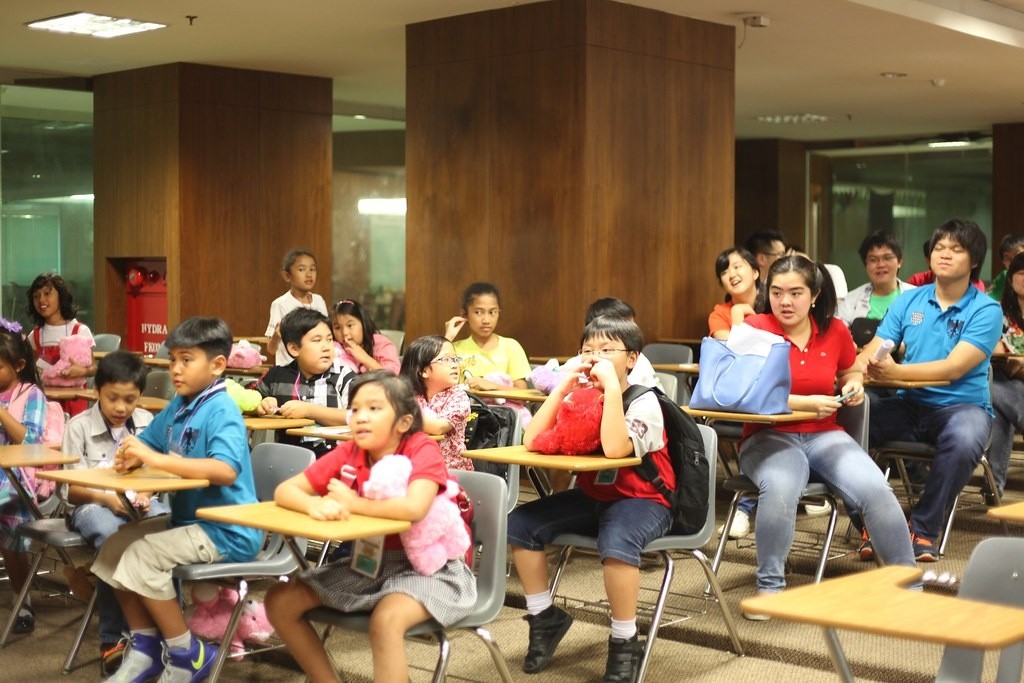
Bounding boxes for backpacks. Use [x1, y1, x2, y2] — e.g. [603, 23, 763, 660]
[9, 381, 65, 501]
[622, 385, 708, 535]
[227, 339, 268, 368]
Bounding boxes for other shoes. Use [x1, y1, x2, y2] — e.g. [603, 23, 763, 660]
[718, 511, 751, 538]
[12, 600, 35, 635]
[601, 629, 644, 683]
[98, 629, 129, 674]
[523, 606, 573, 673]
[806, 499, 835, 516]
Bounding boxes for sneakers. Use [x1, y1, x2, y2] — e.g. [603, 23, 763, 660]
[858, 527, 874, 560]
[102, 632, 164, 683]
[157, 636, 218, 683]
[908, 522, 940, 562]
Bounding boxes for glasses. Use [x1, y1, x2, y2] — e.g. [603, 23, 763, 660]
[577, 347, 632, 358]
[425, 355, 464, 366]
[762, 251, 784, 257]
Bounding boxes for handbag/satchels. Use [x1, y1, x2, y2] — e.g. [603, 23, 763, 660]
[464, 388, 515, 482]
[689, 337, 792, 416]
[849, 317, 885, 347]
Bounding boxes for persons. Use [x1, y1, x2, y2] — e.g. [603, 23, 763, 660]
[708, 218, 1024, 621]
[1, 250, 676, 683]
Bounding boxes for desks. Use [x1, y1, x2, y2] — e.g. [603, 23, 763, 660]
[243, 414, 315, 430]
[657, 337, 702, 345]
[93, 351, 143, 359]
[1, 443, 82, 468]
[43, 388, 91, 398]
[528, 355, 572, 363]
[142, 358, 170, 367]
[650, 362, 700, 373]
[475, 389, 547, 402]
[456, 444, 643, 472]
[233, 335, 269, 345]
[76, 387, 169, 410]
[739, 562, 1024, 650]
[286, 423, 445, 442]
[195, 500, 413, 544]
[34, 461, 209, 492]
[227, 365, 271, 374]
[681, 405, 818, 421]
[865, 379, 951, 388]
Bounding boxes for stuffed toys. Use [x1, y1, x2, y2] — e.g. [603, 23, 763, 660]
[226, 340, 268, 369]
[225, 379, 262, 416]
[525, 354, 586, 399]
[475, 374, 533, 431]
[361, 454, 471, 576]
[532, 376, 606, 456]
[181, 580, 275, 663]
[41, 335, 94, 388]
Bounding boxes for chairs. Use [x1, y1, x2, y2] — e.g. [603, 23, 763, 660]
[1, 331, 1023, 683]
